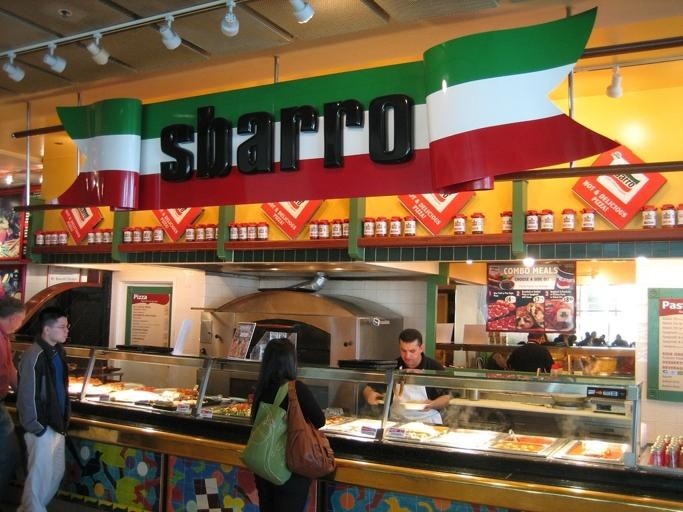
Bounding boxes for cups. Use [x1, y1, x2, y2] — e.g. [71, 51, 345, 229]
[554, 265, 574, 291]
[499, 280, 514, 290]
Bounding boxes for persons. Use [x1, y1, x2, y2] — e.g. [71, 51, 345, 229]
[361, 326, 454, 424]
[250, 337, 328, 512]
[0, 296, 28, 504]
[0, 217, 13, 243]
[15, 305, 72, 512]
[506, 331, 554, 373]
[486, 341, 509, 368]
[516, 327, 630, 348]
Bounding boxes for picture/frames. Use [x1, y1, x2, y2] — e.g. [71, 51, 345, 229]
[645, 286, 682, 404]
[124, 284, 172, 349]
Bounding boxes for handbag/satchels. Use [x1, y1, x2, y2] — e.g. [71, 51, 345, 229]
[239, 401, 292, 486]
[286, 399, 337, 479]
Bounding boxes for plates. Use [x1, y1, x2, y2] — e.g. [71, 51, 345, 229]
[488, 276, 514, 284]
[399, 402, 430, 410]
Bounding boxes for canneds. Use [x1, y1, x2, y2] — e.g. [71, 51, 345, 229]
[34, 230, 68, 247]
[123, 226, 164, 243]
[452, 214, 468, 235]
[363, 216, 416, 237]
[525, 208, 595, 232]
[640, 204, 683, 229]
[185, 223, 219, 242]
[87, 229, 113, 245]
[471, 213, 484, 234]
[500, 211, 512, 233]
[229, 222, 269, 241]
[309, 218, 350, 239]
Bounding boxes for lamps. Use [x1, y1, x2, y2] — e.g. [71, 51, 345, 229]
[605, 65, 623, 98]
[0, 0, 314, 83]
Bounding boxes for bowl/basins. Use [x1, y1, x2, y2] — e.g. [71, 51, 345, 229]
[551, 391, 587, 407]
